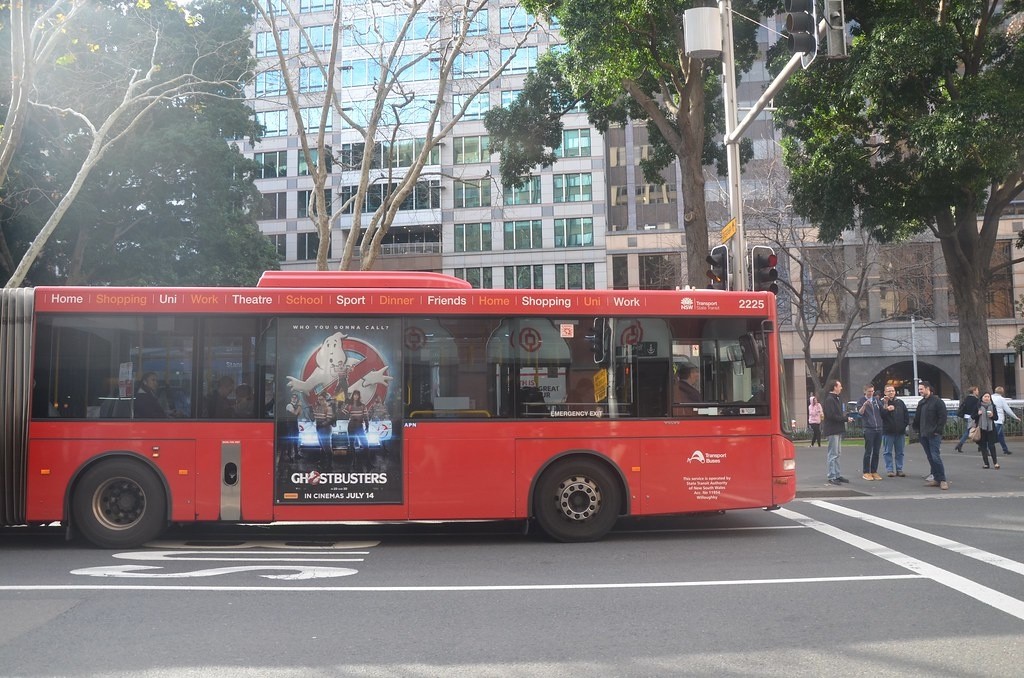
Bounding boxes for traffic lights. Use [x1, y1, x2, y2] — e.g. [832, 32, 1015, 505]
[706, 252, 728, 290]
[757, 251, 779, 296]
[782, 0, 815, 55]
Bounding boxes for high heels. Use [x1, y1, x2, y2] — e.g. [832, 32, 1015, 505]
[977, 446, 981, 452]
[955, 442, 963, 452]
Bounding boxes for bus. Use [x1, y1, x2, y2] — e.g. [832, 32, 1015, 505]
[1, 269, 799, 550]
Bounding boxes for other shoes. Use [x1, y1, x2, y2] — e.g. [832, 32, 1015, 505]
[830, 479, 841, 486]
[939, 480, 948, 490]
[896, 470, 906, 477]
[819, 412, 825, 422]
[808, 444, 814, 448]
[994, 463, 1000, 468]
[928, 480, 939, 486]
[862, 473, 874, 481]
[871, 472, 882, 480]
[1003, 450, 1013, 456]
[887, 471, 895, 476]
[836, 476, 849, 483]
[983, 464, 991, 469]
[922, 474, 934, 482]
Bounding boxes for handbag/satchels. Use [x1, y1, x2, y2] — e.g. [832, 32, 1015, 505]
[957, 400, 967, 418]
[968, 425, 981, 443]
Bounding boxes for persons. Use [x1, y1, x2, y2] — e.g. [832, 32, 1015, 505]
[313, 395, 334, 473]
[955, 386, 980, 453]
[857, 384, 889, 481]
[206, 376, 254, 418]
[678, 363, 703, 403]
[135, 372, 164, 419]
[971, 392, 1001, 469]
[809, 397, 824, 448]
[366, 395, 391, 454]
[911, 381, 949, 490]
[336, 390, 375, 469]
[990, 387, 1021, 454]
[821, 380, 854, 485]
[285, 394, 302, 462]
[880, 384, 909, 478]
